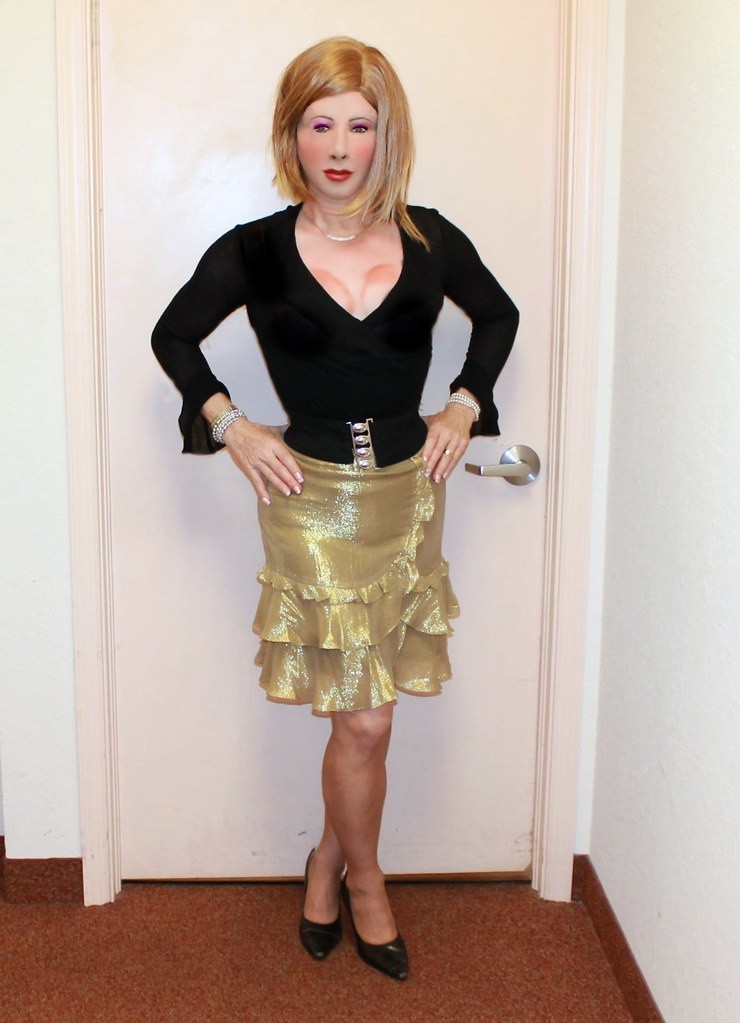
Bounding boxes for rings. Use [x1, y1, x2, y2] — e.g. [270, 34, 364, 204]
[443, 449, 454, 457]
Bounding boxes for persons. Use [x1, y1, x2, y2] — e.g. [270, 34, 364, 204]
[152, 38, 519, 982]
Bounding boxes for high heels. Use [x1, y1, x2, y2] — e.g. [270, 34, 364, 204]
[342, 870, 409, 982]
[299, 848, 343, 961]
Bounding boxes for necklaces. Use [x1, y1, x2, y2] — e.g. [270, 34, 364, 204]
[300, 208, 377, 241]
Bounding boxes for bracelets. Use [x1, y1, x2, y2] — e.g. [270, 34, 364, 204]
[210, 404, 246, 444]
[445, 392, 480, 421]
